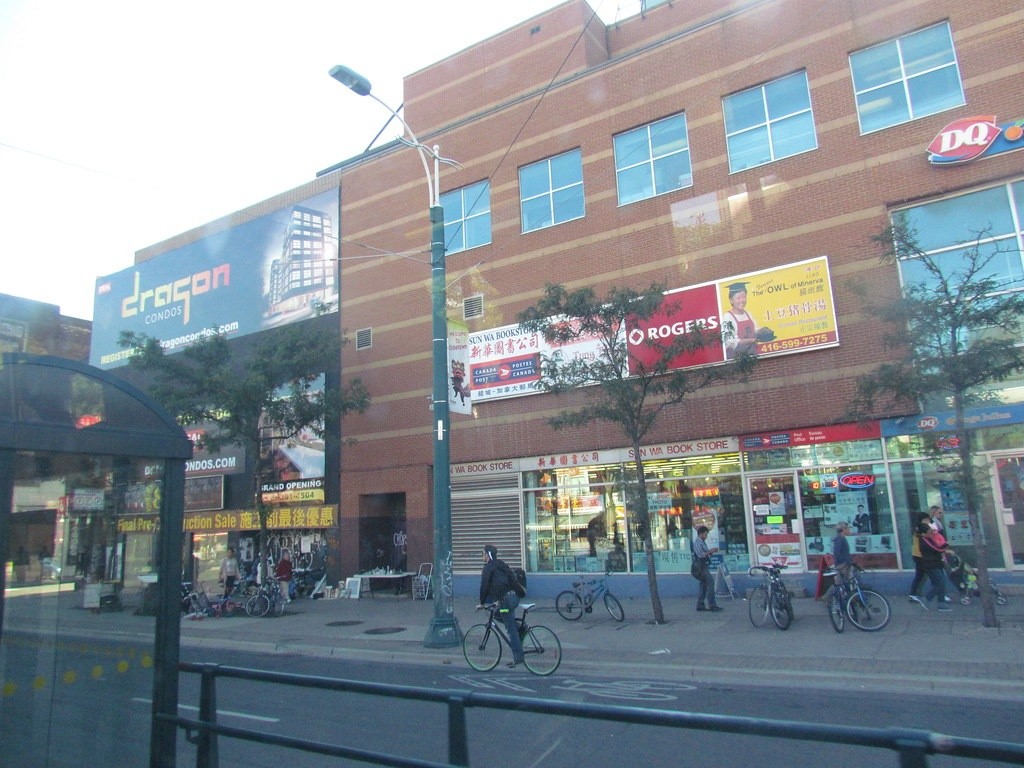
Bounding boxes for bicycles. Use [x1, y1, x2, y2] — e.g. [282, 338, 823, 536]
[820, 561, 891, 633]
[181, 581, 246, 620]
[244, 574, 289, 618]
[555, 568, 625, 623]
[463, 604, 563, 677]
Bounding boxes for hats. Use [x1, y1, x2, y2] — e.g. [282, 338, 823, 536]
[725, 280, 749, 297]
[928, 522, 937, 530]
[483, 544, 497, 561]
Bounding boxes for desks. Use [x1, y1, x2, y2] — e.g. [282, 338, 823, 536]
[353, 568, 416, 600]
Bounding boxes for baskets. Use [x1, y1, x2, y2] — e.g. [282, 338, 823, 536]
[856, 572, 877, 591]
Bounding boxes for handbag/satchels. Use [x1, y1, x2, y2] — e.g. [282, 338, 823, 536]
[512, 568, 526, 598]
[691, 560, 707, 580]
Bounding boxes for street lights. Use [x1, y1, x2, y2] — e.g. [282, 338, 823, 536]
[327, 64, 465, 649]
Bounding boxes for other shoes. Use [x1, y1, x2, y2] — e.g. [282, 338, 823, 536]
[938, 602, 953, 612]
[944, 594, 951, 602]
[506, 659, 524, 667]
[696, 607, 710, 612]
[909, 594, 922, 603]
[711, 606, 724, 611]
[519, 626, 526, 640]
[920, 597, 931, 611]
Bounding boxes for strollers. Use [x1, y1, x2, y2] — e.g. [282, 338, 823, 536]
[942, 550, 1008, 606]
[748, 565, 795, 632]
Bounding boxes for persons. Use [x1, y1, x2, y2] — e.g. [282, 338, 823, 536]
[276, 552, 293, 604]
[853, 505, 870, 534]
[12, 548, 52, 583]
[722, 282, 760, 359]
[218, 548, 241, 602]
[252, 553, 261, 589]
[819, 522, 852, 607]
[694, 527, 721, 611]
[477, 545, 529, 667]
[910, 507, 953, 612]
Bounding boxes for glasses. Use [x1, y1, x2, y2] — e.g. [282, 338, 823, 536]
[938, 511, 942, 515]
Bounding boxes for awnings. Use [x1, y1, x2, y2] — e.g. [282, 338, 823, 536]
[526, 513, 597, 530]
